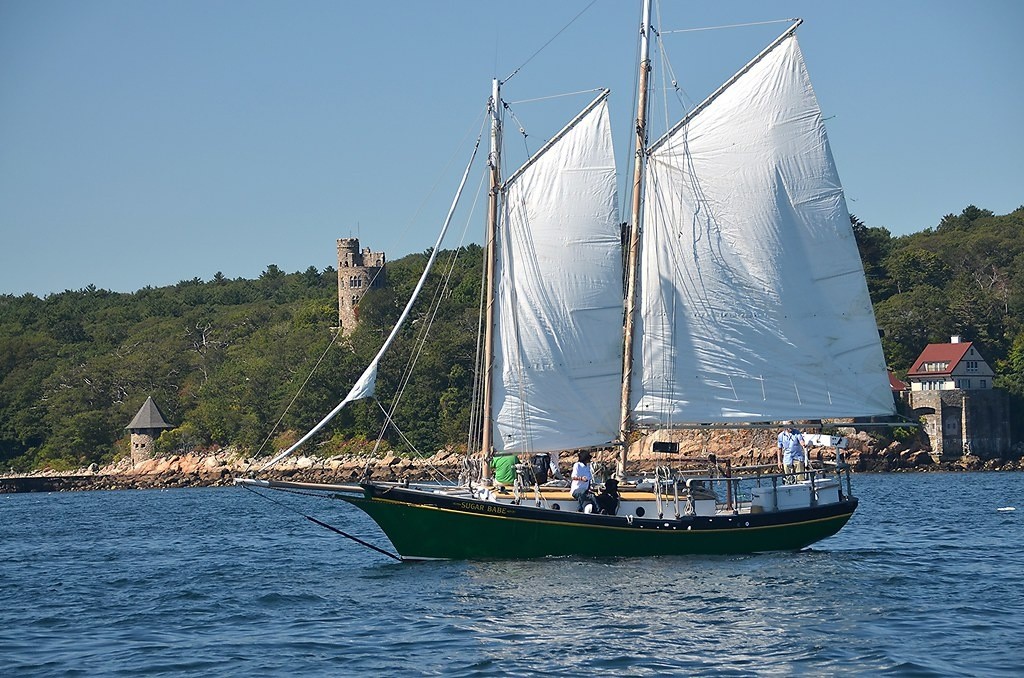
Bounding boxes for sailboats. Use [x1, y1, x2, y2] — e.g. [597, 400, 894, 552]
[228, 0, 918, 565]
[432, 472, 717, 513]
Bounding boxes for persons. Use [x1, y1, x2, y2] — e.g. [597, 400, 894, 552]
[777, 420, 808, 485]
[548, 452, 563, 481]
[490, 453, 521, 487]
[571, 450, 606, 515]
